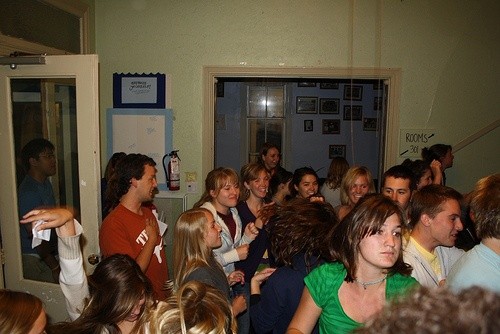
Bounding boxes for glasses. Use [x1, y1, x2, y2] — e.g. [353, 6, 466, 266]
[40, 154, 56, 160]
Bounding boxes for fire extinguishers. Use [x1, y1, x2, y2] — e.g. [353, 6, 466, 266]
[161, 148, 182, 192]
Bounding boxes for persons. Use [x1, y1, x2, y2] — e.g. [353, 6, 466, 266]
[286, 192, 420, 334]
[251, 202, 339, 334]
[195, 167, 258, 277]
[380, 165, 414, 209]
[270, 171, 292, 201]
[98, 153, 168, 301]
[293, 166, 324, 201]
[173, 208, 246, 334]
[412, 159, 433, 189]
[0, 289, 47, 334]
[446, 174, 500, 299]
[256, 145, 287, 179]
[454, 191, 482, 251]
[18, 139, 63, 282]
[137, 279, 237, 334]
[47, 254, 152, 334]
[421, 144, 454, 187]
[337, 166, 375, 216]
[404, 184, 469, 296]
[321, 156, 351, 206]
[235, 163, 275, 246]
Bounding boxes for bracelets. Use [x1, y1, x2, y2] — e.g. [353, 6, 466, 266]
[52, 264, 60, 272]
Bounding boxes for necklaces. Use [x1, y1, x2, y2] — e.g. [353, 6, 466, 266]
[353, 273, 388, 288]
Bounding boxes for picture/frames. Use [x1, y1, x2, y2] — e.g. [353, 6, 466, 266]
[294, 82, 381, 159]
[106, 109, 173, 191]
